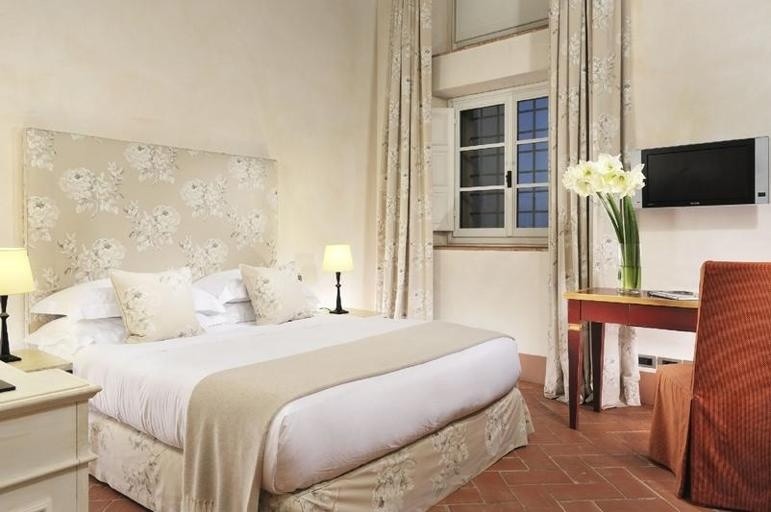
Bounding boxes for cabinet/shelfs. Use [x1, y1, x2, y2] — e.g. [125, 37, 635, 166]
[0, 360, 103, 512]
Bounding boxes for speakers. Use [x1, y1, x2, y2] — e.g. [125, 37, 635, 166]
[755, 135, 770, 205]
[630, 149, 642, 209]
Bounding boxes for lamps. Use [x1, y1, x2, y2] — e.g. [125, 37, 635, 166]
[0, 247, 37, 362]
[323, 243, 351, 314]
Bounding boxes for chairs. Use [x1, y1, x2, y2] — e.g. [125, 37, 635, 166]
[645, 261, 771, 512]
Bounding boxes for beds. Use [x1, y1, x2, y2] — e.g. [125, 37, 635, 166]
[20, 126, 534, 512]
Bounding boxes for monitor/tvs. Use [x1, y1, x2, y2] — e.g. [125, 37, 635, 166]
[641, 137, 756, 209]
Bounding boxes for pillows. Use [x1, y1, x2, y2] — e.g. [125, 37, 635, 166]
[24, 314, 223, 347]
[107, 269, 203, 342]
[238, 262, 321, 327]
[192, 268, 251, 303]
[224, 299, 257, 323]
[29, 274, 224, 322]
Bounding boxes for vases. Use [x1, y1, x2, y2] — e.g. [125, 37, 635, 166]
[616, 241, 642, 298]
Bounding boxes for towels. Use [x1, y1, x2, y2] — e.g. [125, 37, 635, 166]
[181, 319, 513, 512]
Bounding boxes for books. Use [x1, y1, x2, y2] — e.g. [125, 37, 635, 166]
[647, 289, 699, 301]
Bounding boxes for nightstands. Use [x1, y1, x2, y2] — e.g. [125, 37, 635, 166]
[10, 347, 73, 374]
[337, 308, 387, 316]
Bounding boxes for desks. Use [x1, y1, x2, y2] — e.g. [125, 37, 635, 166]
[562, 288, 699, 429]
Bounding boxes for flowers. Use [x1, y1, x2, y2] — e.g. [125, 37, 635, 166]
[561, 152, 647, 290]
